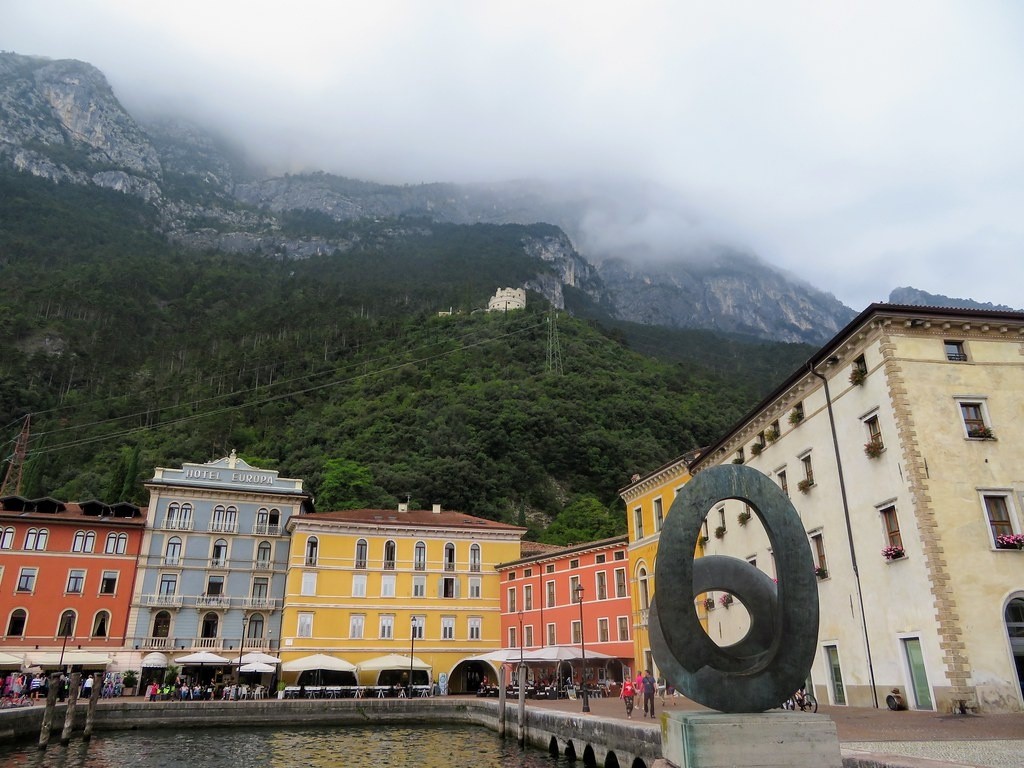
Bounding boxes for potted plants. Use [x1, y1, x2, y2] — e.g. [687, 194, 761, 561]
[863, 442, 883, 459]
[787, 409, 804, 427]
[698, 536, 709, 548]
[750, 442, 765, 455]
[715, 527, 726, 538]
[277, 679, 288, 699]
[764, 428, 780, 443]
[798, 479, 813, 495]
[121, 670, 139, 695]
[849, 368, 866, 387]
[737, 512, 750, 527]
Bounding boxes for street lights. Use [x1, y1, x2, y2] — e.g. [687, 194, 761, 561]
[408, 615, 418, 699]
[516, 608, 524, 664]
[574, 584, 591, 712]
[234, 615, 249, 701]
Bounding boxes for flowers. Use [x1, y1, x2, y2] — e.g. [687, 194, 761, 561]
[881, 545, 905, 560]
[703, 598, 713, 611]
[815, 565, 827, 578]
[772, 578, 778, 583]
[971, 425, 992, 437]
[997, 534, 1024, 550]
[717, 594, 732, 609]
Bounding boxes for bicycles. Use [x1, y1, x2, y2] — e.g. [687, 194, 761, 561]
[0, 682, 34, 710]
[781, 689, 818, 713]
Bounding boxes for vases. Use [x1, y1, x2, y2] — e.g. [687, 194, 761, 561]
[999, 543, 1017, 549]
[728, 600, 733, 603]
[968, 431, 991, 438]
[710, 604, 714, 608]
[821, 571, 828, 579]
[891, 552, 904, 559]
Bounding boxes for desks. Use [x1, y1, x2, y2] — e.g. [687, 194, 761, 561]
[477, 684, 602, 700]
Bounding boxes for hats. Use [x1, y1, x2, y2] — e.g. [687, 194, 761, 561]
[88, 675, 93, 678]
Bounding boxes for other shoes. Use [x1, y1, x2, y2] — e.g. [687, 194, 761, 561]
[634, 705, 639, 710]
[36, 699, 40, 701]
[651, 715, 655, 718]
[644, 712, 647, 717]
[628, 716, 631, 719]
[642, 708, 644, 710]
[662, 700, 665, 706]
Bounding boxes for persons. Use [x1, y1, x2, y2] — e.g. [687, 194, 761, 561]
[0, 672, 263, 701]
[477, 669, 679, 718]
[795, 690, 807, 711]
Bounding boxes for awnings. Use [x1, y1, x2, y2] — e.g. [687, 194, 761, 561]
[0, 653, 25, 665]
[24, 652, 112, 668]
[141, 652, 168, 668]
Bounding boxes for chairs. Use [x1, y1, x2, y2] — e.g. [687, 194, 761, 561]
[240, 684, 270, 700]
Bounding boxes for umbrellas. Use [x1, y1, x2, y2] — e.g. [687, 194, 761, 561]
[237, 661, 276, 673]
[173, 651, 231, 666]
[506, 645, 617, 697]
[230, 651, 282, 665]
[468, 650, 531, 664]
[356, 654, 432, 671]
[281, 653, 358, 686]
